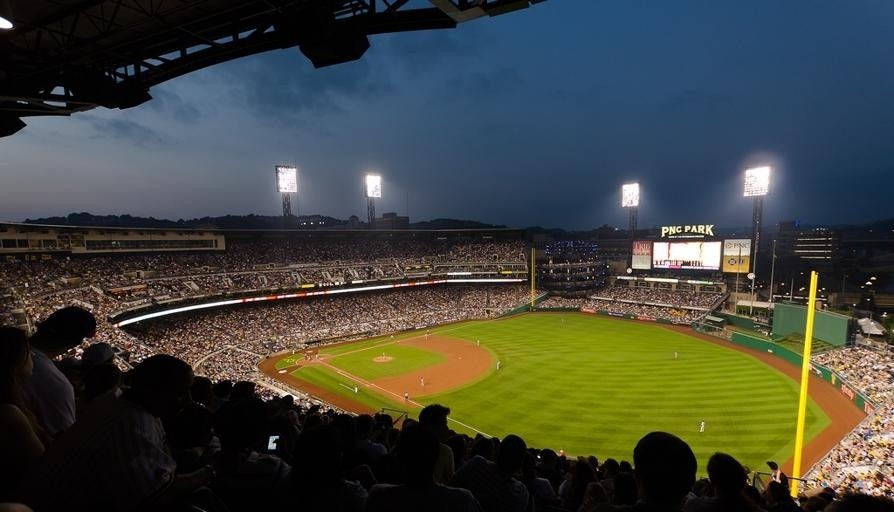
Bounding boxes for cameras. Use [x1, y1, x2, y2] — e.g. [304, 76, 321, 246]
[267, 435, 280, 451]
